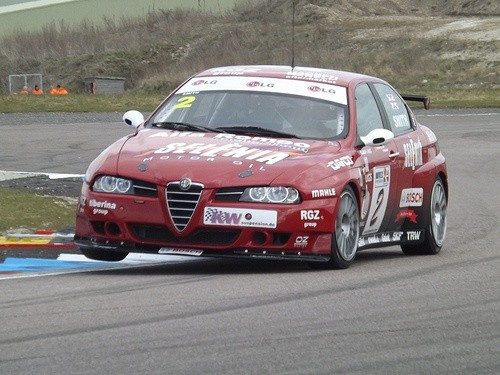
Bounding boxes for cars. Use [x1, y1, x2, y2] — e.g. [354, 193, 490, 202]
[74, 64, 449, 270]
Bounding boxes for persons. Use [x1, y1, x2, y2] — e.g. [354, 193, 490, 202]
[298, 105, 332, 137]
